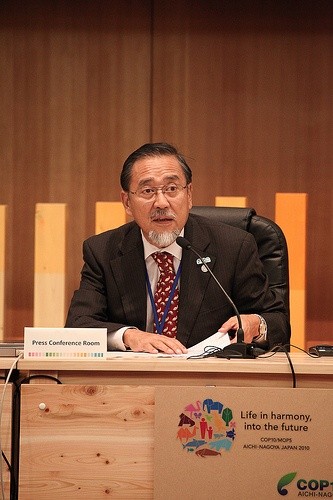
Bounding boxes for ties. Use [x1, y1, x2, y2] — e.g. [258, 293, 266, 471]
[152, 250, 181, 339]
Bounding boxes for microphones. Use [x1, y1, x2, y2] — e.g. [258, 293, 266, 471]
[176, 236, 257, 359]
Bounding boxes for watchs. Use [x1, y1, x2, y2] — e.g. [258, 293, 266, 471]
[251, 314, 267, 342]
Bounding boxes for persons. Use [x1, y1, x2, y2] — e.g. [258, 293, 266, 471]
[64, 143, 292, 354]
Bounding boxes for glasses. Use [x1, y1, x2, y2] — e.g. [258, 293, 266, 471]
[126, 183, 188, 197]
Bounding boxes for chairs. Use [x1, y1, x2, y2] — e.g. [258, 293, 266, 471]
[190, 206, 291, 353]
